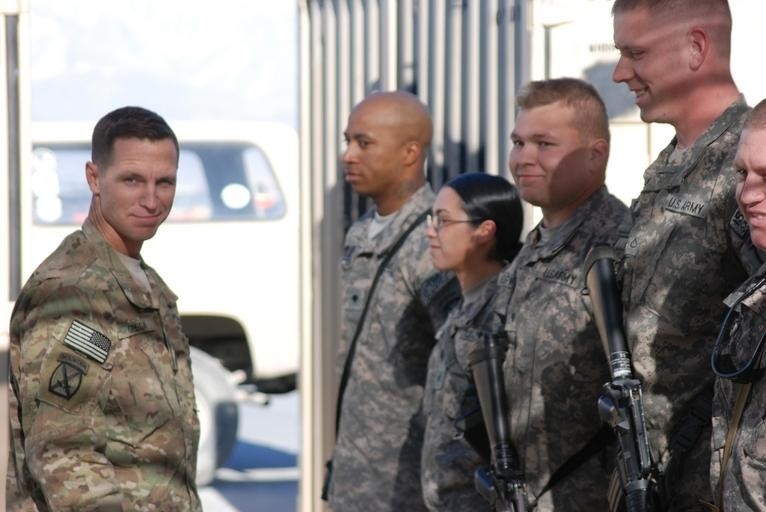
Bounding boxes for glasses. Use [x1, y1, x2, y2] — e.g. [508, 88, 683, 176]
[426, 214, 472, 230]
[711, 278, 766, 384]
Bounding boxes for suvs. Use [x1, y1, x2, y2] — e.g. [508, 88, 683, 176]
[19, 114, 299, 488]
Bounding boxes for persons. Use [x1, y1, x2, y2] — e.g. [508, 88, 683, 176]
[608, 0, 766, 512]
[327, 90, 462, 512]
[490, 79, 628, 512]
[420, 174, 523, 512]
[710, 99, 766, 512]
[5, 107, 203, 512]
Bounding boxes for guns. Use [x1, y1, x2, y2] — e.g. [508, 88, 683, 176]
[467, 333, 530, 511]
[581, 243, 658, 511]
